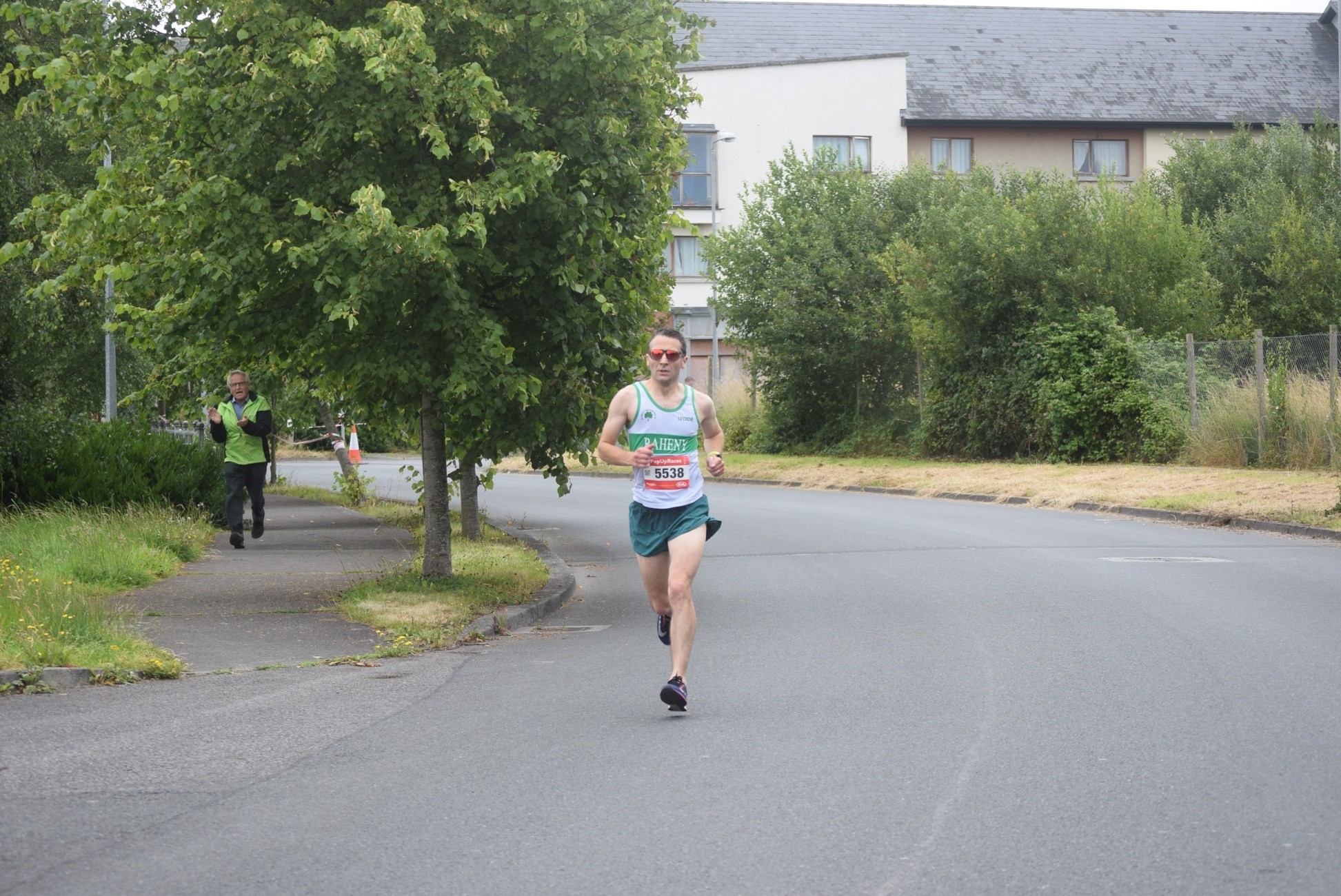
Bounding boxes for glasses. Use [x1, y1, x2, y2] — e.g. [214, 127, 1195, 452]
[648, 349, 682, 361]
[228, 382, 246, 387]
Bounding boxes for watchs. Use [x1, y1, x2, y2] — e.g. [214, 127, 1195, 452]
[708, 451, 722, 459]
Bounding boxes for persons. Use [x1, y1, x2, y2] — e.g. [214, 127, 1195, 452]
[209, 370, 272, 549]
[598, 330, 726, 711]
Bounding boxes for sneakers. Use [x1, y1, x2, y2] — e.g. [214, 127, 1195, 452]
[251, 518, 264, 538]
[230, 531, 244, 548]
[660, 675, 688, 712]
[657, 615, 671, 645]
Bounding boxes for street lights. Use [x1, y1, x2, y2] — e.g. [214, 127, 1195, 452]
[708, 135, 738, 407]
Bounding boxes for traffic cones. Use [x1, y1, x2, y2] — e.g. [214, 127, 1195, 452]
[348, 425, 367, 468]
